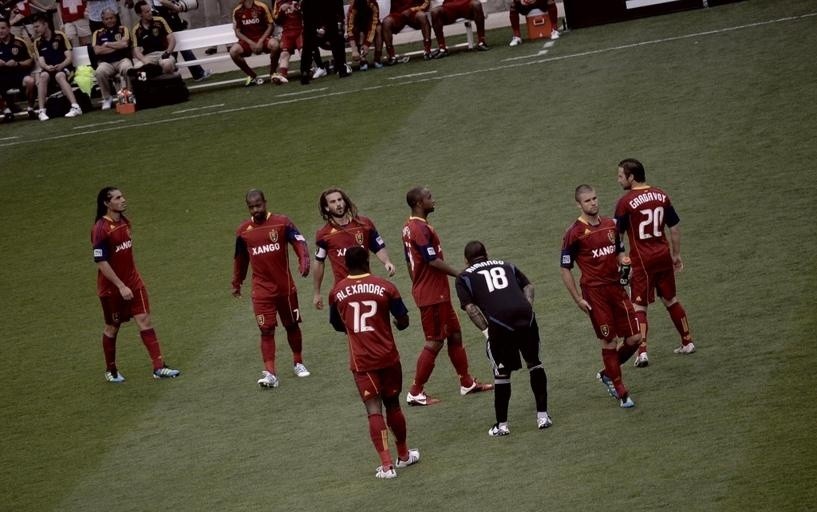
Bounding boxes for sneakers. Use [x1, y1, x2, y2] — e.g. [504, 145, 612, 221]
[673, 342, 696, 354]
[476, 41, 490, 51]
[633, 351, 649, 367]
[488, 421, 511, 437]
[386, 56, 397, 65]
[432, 48, 448, 59]
[395, 448, 420, 468]
[244, 71, 257, 87]
[617, 391, 635, 408]
[596, 367, 618, 401]
[152, 366, 181, 379]
[2, 113, 16, 123]
[270, 73, 288, 85]
[343, 63, 352, 74]
[460, 377, 494, 396]
[406, 391, 440, 406]
[193, 71, 211, 82]
[104, 370, 127, 383]
[423, 50, 433, 61]
[301, 70, 309, 85]
[65, 107, 83, 117]
[359, 64, 368, 71]
[509, 36, 522, 47]
[535, 415, 553, 429]
[102, 95, 113, 110]
[38, 111, 50, 121]
[257, 370, 279, 388]
[375, 465, 398, 479]
[374, 61, 383, 68]
[293, 362, 311, 378]
[312, 66, 327, 79]
[28, 111, 36, 120]
[550, 28, 559, 40]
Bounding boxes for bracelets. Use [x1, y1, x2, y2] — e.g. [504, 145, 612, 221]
[576, 296, 583, 304]
[481, 326, 491, 341]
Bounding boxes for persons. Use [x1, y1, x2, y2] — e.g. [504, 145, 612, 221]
[230, 1, 561, 88]
[311, 187, 395, 311]
[453, 240, 553, 438]
[400, 186, 494, 406]
[558, 183, 642, 407]
[229, 189, 311, 388]
[88, 186, 180, 384]
[0, 0, 233, 122]
[328, 246, 420, 480]
[608, 158, 696, 367]
